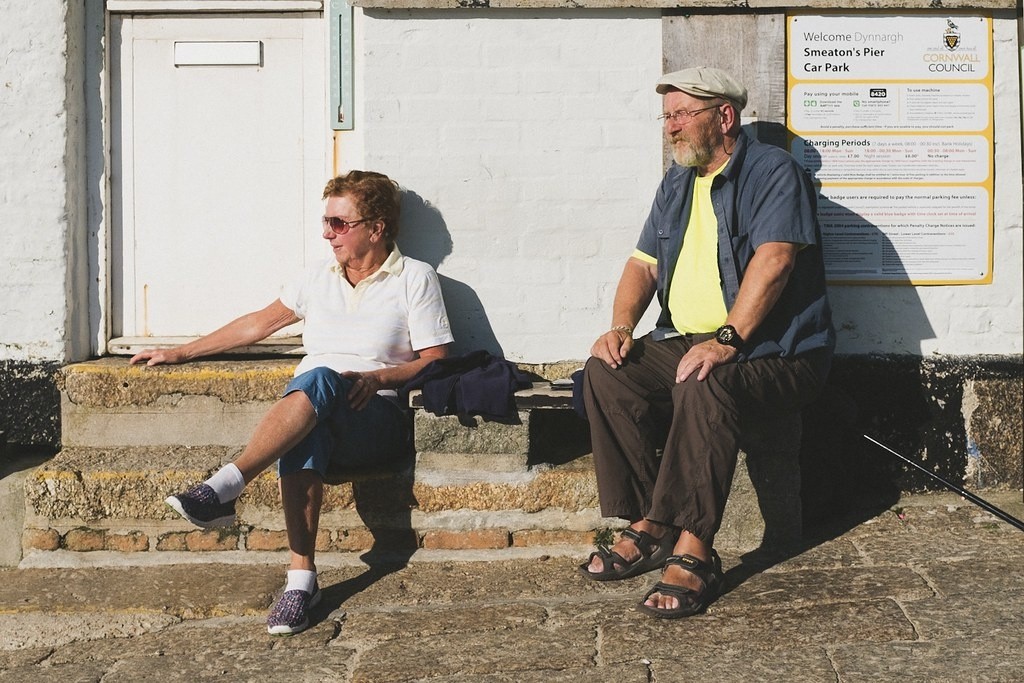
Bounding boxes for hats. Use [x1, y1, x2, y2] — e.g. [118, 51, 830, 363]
[655, 66, 748, 112]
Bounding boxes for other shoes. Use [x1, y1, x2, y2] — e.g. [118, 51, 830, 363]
[164, 481, 237, 528]
[267, 575, 321, 634]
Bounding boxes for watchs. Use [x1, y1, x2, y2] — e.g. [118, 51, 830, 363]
[715, 325, 745, 351]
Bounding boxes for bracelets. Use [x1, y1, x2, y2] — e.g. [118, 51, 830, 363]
[610, 326, 632, 336]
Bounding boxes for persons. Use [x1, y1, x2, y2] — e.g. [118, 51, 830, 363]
[578, 64, 838, 619]
[129, 168, 454, 635]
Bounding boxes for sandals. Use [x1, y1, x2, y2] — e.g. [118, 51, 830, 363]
[636, 546, 727, 620]
[581, 527, 678, 581]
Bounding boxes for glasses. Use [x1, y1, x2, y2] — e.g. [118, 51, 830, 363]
[321, 215, 379, 234]
[658, 104, 724, 126]
